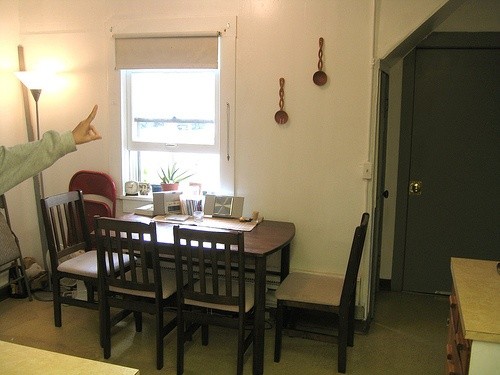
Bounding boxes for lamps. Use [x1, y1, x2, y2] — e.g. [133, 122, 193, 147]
[17, 71, 55, 300]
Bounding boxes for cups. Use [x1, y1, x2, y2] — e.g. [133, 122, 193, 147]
[194, 211, 204, 223]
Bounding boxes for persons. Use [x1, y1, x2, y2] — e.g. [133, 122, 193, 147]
[0, 104, 102, 197]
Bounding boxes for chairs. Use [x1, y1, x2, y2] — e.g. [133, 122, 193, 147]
[42, 171, 253, 375]
[0, 195, 33, 301]
[275, 212, 370, 372]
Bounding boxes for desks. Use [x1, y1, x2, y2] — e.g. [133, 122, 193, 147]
[90, 213, 296, 375]
[0, 340, 140, 375]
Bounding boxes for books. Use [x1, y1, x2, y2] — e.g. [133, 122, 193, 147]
[133, 204, 153, 216]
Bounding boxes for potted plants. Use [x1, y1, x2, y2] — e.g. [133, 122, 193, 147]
[158, 160, 194, 191]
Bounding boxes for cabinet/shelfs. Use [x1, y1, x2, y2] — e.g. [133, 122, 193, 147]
[445, 257, 500, 375]
